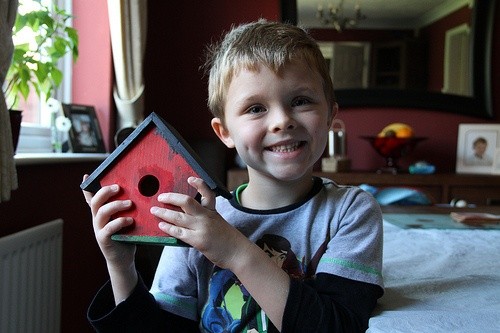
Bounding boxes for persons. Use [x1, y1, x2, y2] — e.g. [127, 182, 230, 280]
[465, 137, 493, 167]
[80, 20, 384, 333]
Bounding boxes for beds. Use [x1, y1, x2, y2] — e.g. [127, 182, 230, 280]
[228, 169, 500, 333]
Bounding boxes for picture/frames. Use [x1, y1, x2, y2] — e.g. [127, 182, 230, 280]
[60, 103, 107, 153]
[456, 123, 500, 174]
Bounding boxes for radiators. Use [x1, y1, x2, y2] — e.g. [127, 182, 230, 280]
[0, 218, 63, 333]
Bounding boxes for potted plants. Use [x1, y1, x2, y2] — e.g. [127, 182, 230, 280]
[4, 0, 80, 154]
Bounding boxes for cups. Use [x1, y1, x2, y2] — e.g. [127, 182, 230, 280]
[329, 128, 348, 158]
